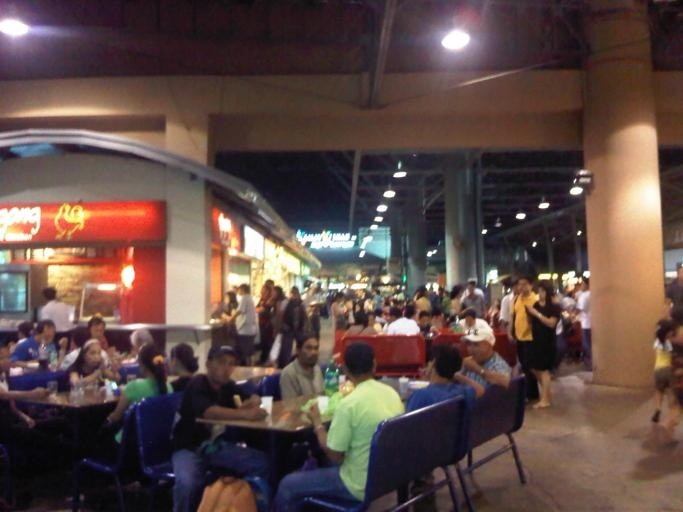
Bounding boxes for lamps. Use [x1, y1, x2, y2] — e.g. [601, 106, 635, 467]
[357, 161, 593, 258]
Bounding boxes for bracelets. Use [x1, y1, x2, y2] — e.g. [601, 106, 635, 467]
[310, 424, 326, 435]
[479, 367, 486, 377]
[537, 313, 542, 319]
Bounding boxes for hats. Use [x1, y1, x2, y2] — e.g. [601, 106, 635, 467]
[460, 328, 496, 346]
[208, 346, 238, 361]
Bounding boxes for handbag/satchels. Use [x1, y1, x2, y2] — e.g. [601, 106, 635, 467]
[562, 318, 576, 336]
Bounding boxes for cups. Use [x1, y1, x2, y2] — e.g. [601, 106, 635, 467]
[71, 380, 87, 400]
[399, 378, 408, 398]
[46, 378, 56, 396]
[260, 394, 272, 416]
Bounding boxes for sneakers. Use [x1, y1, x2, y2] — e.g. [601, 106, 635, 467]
[533, 399, 551, 408]
[652, 410, 660, 422]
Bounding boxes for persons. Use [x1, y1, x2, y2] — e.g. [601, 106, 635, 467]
[211, 274, 591, 411]
[407, 345, 469, 413]
[648, 318, 677, 425]
[452, 321, 518, 396]
[2, 285, 199, 512]
[167, 343, 272, 512]
[665, 261, 683, 331]
[273, 340, 406, 512]
[279, 332, 327, 402]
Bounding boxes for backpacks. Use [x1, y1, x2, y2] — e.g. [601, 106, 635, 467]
[197, 477, 257, 511]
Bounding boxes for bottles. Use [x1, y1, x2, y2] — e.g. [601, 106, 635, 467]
[453, 315, 462, 333]
[323, 360, 339, 394]
[37, 339, 50, 368]
